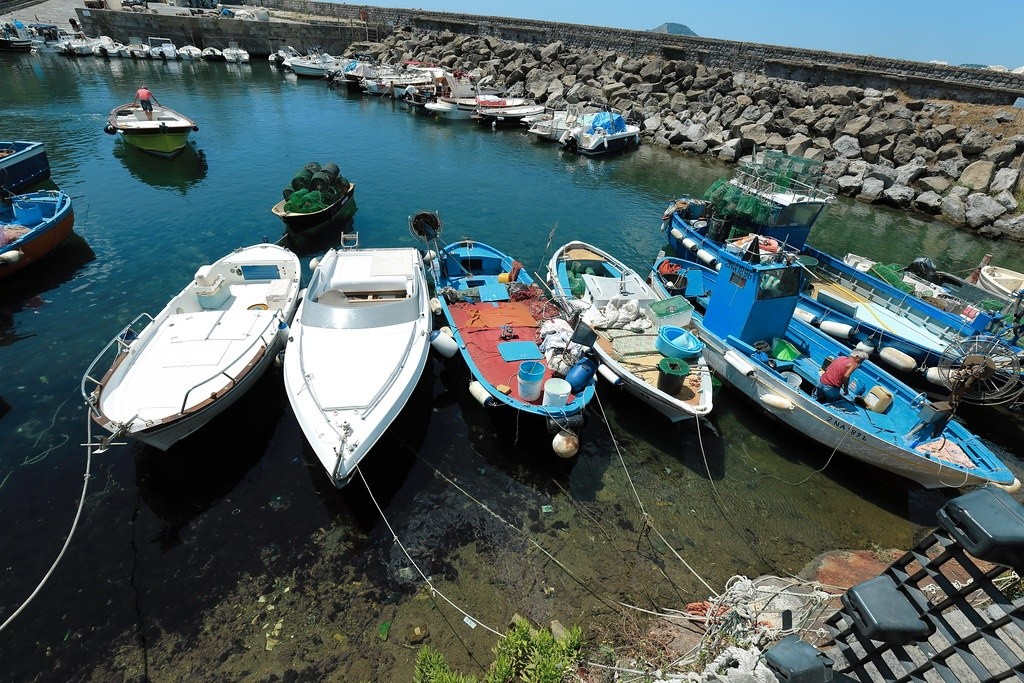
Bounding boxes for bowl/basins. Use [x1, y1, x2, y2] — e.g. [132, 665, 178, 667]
[656, 325, 705, 360]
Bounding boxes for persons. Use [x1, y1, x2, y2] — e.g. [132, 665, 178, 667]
[134, 85, 162, 121]
[812, 349, 870, 403]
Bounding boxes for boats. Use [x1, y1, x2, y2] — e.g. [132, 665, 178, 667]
[268, 46, 642, 155]
[201, 47, 223, 61]
[660, 193, 1024, 408]
[0, 139, 51, 193]
[908, 257, 1024, 321]
[546, 241, 713, 423]
[271, 180, 356, 228]
[179, 44, 202, 60]
[149, 37, 181, 60]
[964, 254, 1024, 305]
[646, 249, 1021, 494]
[118, 35, 151, 58]
[428, 236, 596, 426]
[0, 19, 125, 57]
[843, 251, 1023, 349]
[0, 189, 75, 275]
[276, 232, 433, 488]
[79, 233, 301, 452]
[107, 102, 200, 151]
[221, 40, 250, 62]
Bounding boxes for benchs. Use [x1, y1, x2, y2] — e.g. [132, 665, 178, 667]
[330, 275, 408, 303]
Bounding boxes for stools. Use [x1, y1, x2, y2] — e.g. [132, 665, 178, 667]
[266, 278, 292, 310]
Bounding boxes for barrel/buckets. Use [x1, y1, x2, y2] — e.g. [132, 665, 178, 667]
[661, 273, 688, 297]
[671, 332, 694, 349]
[864, 385, 893, 412]
[848, 376, 866, 394]
[781, 371, 802, 390]
[656, 356, 691, 396]
[678, 199, 705, 219]
[517, 357, 597, 407]
[920, 294, 969, 317]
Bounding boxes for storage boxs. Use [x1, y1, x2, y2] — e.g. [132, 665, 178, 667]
[646, 295, 694, 332]
[194, 278, 231, 309]
[195, 265, 218, 286]
